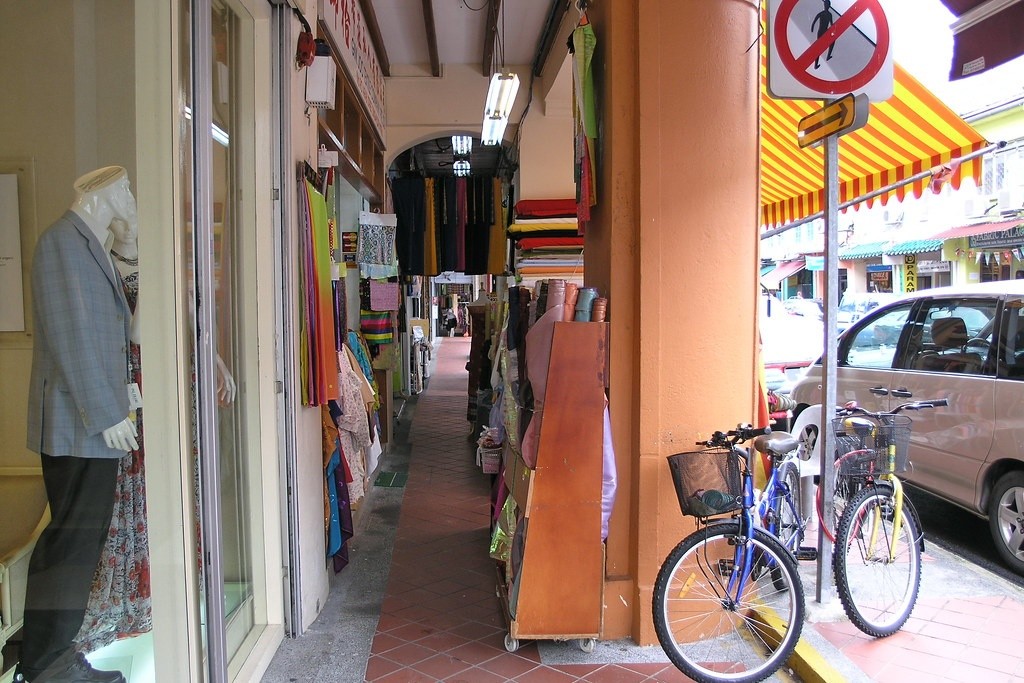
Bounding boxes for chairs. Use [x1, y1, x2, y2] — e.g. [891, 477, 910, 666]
[915, 316, 982, 374]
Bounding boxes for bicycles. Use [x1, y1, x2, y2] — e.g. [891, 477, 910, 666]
[831, 397, 949, 638]
[651, 425, 820, 683]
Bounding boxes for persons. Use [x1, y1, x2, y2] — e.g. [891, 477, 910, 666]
[13, 165, 236, 683]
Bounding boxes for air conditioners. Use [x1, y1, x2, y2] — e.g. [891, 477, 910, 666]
[998, 187, 1024, 215]
[882, 206, 903, 225]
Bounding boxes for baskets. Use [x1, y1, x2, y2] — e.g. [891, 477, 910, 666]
[480, 445, 502, 474]
[831, 413, 913, 475]
[666, 449, 744, 517]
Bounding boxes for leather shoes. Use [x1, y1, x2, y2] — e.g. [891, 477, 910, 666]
[12, 643, 126, 683]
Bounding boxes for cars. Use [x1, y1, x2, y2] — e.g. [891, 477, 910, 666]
[760, 291, 996, 423]
[788, 278, 1024, 579]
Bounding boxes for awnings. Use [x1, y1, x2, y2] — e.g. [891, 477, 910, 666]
[760, 260, 806, 290]
[759, 0, 1024, 231]
[839, 238, 944, 260]
[925, 219, 1023, 241]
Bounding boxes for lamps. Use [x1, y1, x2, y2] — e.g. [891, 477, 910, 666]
[479, 0, 520, 147]
[451, 135, 472, 155]
[452, 157, 472, 178]
